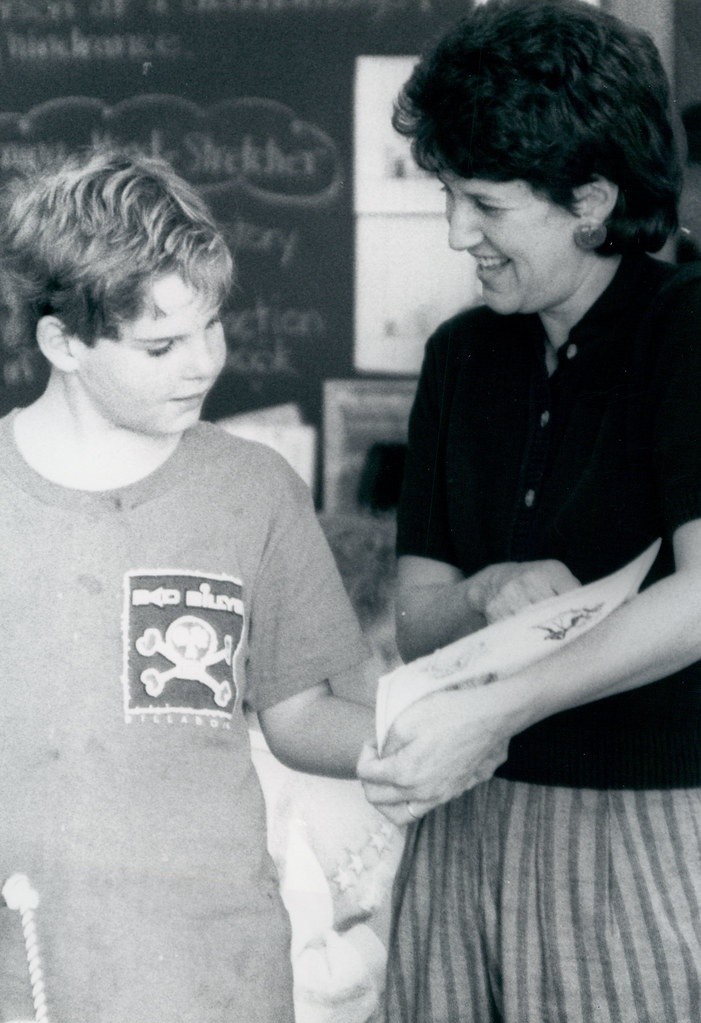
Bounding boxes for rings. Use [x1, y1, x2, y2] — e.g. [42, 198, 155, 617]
[407, 802, 423, 820]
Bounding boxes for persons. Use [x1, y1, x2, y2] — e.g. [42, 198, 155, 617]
[0, 154, 507, 1023]
[353, 0, 700, 1022]
